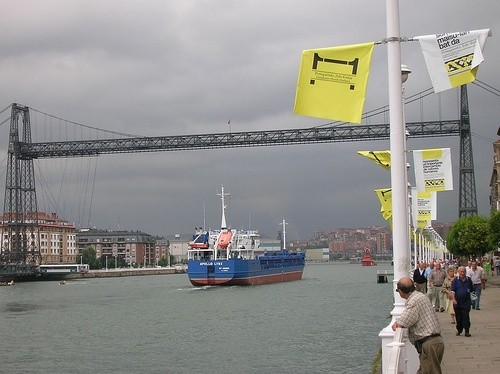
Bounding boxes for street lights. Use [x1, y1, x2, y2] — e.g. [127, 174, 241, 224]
[378, 2, 454, 373]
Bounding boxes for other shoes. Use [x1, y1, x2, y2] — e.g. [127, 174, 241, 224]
[455, 331, 460, 335]
[465, 333, 471, 336]
[435, 307, 439, 311]
[451, 320, 455, 324]
[440, 307, 444, 312]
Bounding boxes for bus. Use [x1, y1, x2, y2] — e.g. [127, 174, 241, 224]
[39, 264, 90, 273]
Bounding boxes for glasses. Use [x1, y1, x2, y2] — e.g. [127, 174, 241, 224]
[396, 289, 398, 291]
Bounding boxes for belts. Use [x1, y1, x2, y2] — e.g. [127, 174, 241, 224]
[433, 284, 441, 286]
[473, 283, 481, 285]
[414, 333, 440, 344]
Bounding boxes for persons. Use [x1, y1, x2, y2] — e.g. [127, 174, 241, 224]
[468, 261, 482, 310]
[429, 262, 446, 312]
[451, 267, 473, 337]
[392, 277, 444, 374]
[441, 267, 454, 324]
[424, 262, 435, 308]
[413, 263, 426, 294]
[409, 257, 500, 290]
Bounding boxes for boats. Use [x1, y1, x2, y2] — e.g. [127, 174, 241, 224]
[361, 247, 379, 266]
[186, 184, 306, 287]
